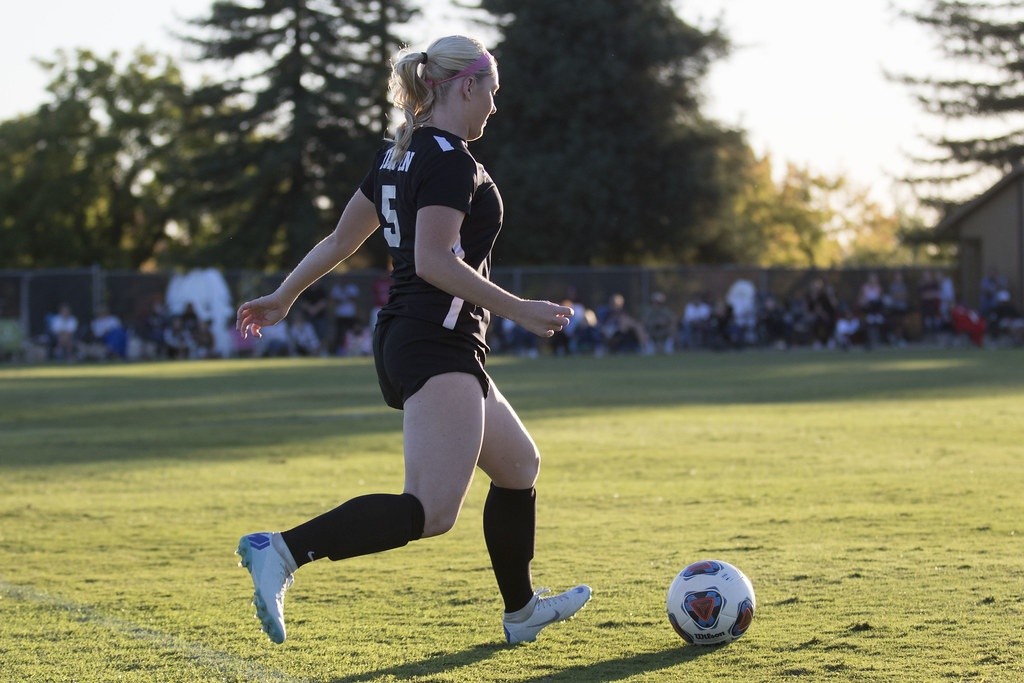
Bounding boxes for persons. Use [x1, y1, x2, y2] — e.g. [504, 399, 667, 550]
[236, 35, 591, 645]
[47, 271, 1024, 368]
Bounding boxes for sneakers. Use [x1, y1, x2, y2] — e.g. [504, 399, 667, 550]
[502, 585, 592, 645]
[234, 531, 296, 645]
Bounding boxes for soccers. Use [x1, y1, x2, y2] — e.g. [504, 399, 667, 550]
[668, 561, 757, 648]
